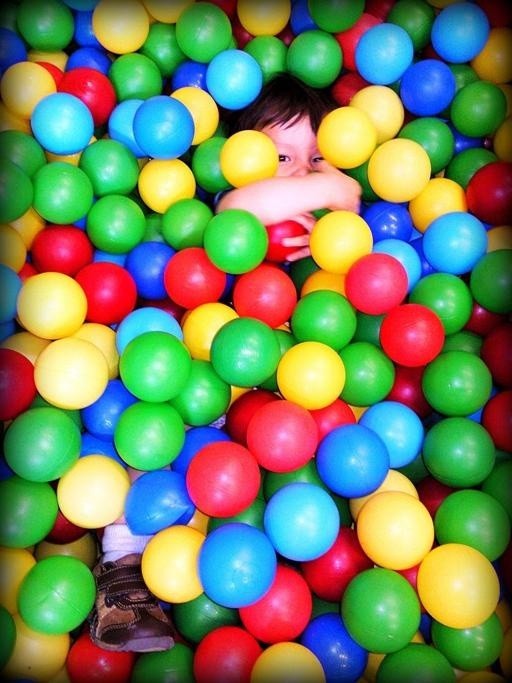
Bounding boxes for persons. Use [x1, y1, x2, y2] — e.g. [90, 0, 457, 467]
[88, 80, 368, 653]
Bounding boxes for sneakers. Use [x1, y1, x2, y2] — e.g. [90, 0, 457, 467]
[89, 555, 175, 653]
